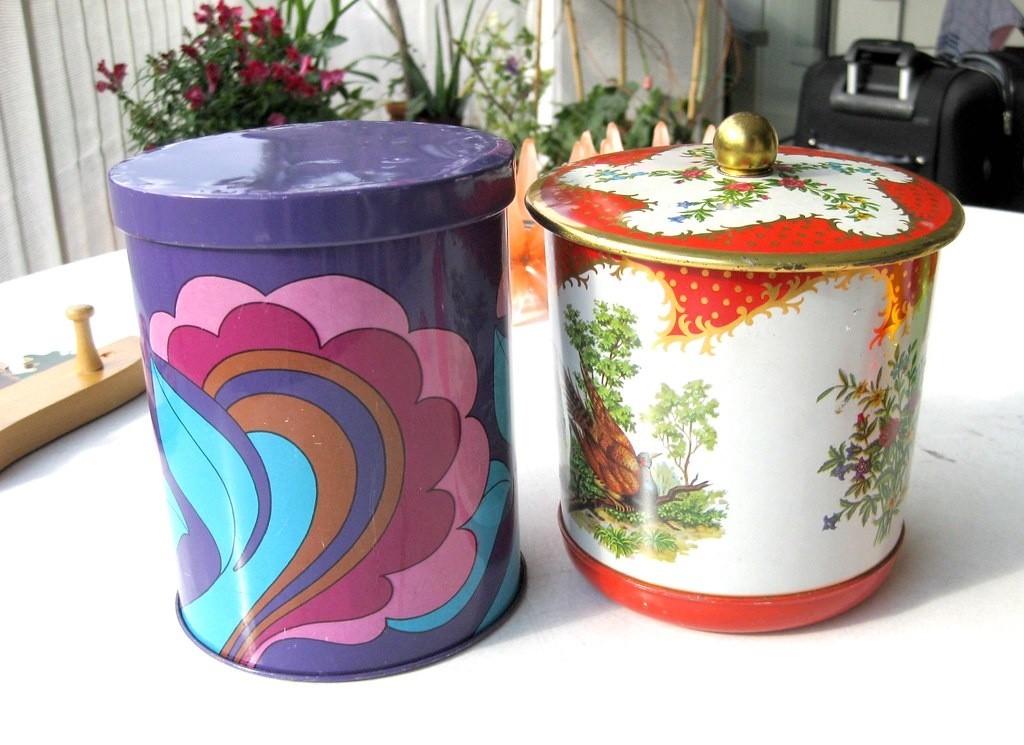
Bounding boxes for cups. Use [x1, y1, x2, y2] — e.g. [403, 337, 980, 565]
[106, 118, 530, 684]
[524, 111, 966, 636]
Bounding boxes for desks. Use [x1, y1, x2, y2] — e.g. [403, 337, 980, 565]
[0, 204, 1024, 745]
[723, 30, 769, 120]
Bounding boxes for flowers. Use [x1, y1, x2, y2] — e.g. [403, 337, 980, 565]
[96, 0, 717, 311]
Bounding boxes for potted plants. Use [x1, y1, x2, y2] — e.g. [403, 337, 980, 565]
[383, 97, 464, 127]
